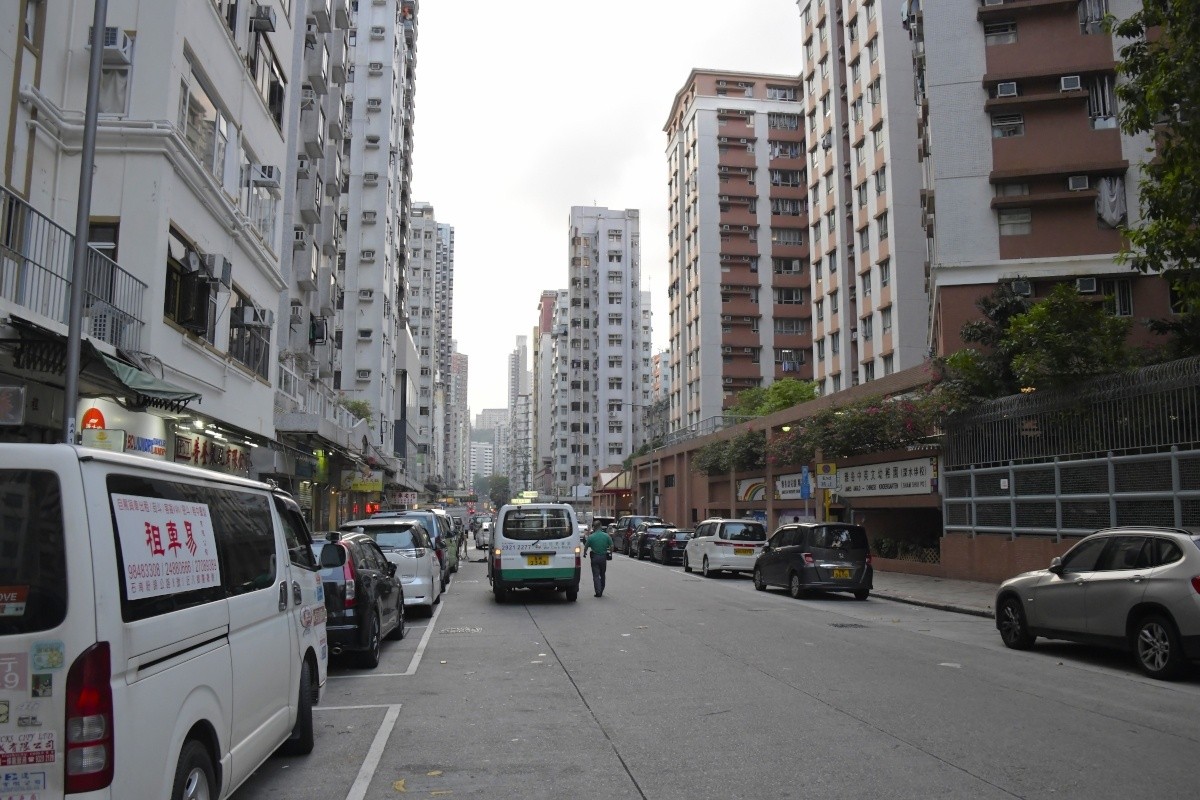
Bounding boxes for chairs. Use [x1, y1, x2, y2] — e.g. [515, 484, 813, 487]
[1125, 548, 1148, 568]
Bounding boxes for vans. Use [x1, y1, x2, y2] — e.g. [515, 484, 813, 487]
[609, 515, 663, 556]
[681, 516, 768, 577]
[486, 502, 582, 604]
[0, 441, 350, 800]
[750, 521, 874, 601]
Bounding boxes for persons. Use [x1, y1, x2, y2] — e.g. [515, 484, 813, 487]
[583, 521, 613, 597]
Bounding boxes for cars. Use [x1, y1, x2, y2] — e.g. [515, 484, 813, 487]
[472, 514, 491, 536]
[576, 516, 617, 542]
[336, 518, 448, 618]
[647, 528, 695, 565]
[474, 519, 496, 549]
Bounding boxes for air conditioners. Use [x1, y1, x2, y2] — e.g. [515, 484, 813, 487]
[292, 306, 303, 320]
[984, 0, 1002, 6]
[997, 82, 1018, 97]
[198, 252, 231, 288]
[88, 301, 128, 348]
[83, 25, 131, 64]
[303, 89, 316, 106]
[297, 160, 311, 174]
[1076, 278, 1096, 292]
[250, 4, 276, 32]
[1060, 76, 1080, 89]
[1010, 281, 1032, 295]
[307, 24, 319, 40]
[230, 306, 273, 329]
[359, 28, 384, 378]
[252, 165, 280, 187]
[718, 80, 858, 384]
[1067, 176, 1089, 191]
[292, 231, 307, 246]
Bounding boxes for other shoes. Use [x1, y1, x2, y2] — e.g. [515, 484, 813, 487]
[594, 592, 602, 597]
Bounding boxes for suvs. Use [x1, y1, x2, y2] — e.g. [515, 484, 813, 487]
[371, 508, 464, 592]
[308, 532, 407, 669]
[992, 527, 1200, 677]
[628, 521, 678, 562]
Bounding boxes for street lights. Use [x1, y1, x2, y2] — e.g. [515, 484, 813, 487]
[606, 401, 655, 518]
